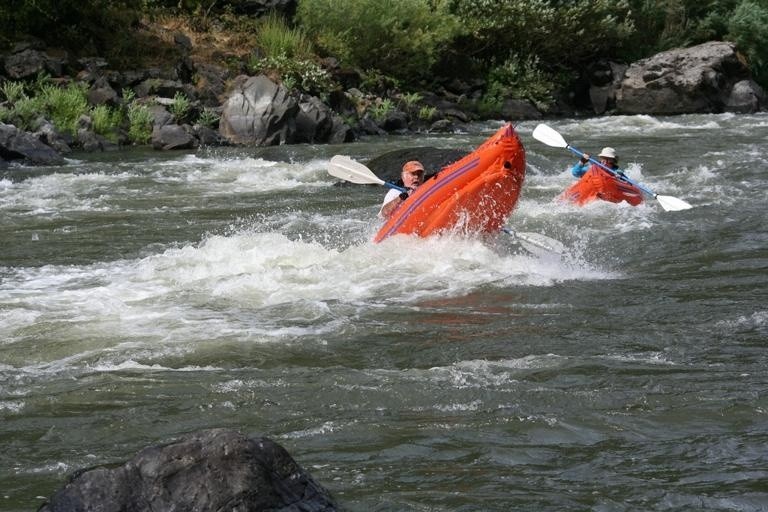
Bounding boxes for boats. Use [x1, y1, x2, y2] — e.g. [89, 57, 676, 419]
[551, 165, 644, 207]
[374, 123, 527, 247]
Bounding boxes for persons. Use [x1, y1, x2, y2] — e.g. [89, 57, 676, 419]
[375, 160, 426, 221]
[572, 147, 632, 186]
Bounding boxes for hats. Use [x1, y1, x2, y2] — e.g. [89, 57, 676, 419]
[597, 147, 616, 158]
[403, 161, 425, 172]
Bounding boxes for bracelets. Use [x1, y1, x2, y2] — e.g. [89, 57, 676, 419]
[580, 157, 588, 164]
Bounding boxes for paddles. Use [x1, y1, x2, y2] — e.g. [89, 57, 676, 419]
[327, 154, 564, 263]
[532, 124, 692, 211]
